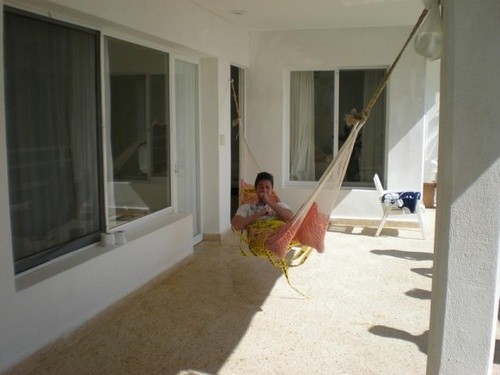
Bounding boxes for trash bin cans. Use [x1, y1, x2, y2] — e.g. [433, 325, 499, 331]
[423, 183, 435, 209]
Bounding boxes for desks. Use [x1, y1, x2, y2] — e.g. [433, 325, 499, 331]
[424, 180, 438, 208]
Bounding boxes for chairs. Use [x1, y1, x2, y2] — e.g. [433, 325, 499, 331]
[373, 174, 427, 240]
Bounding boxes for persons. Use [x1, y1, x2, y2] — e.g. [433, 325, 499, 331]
[232, 171, 324, 257]
[344, 123, 362, 182]
